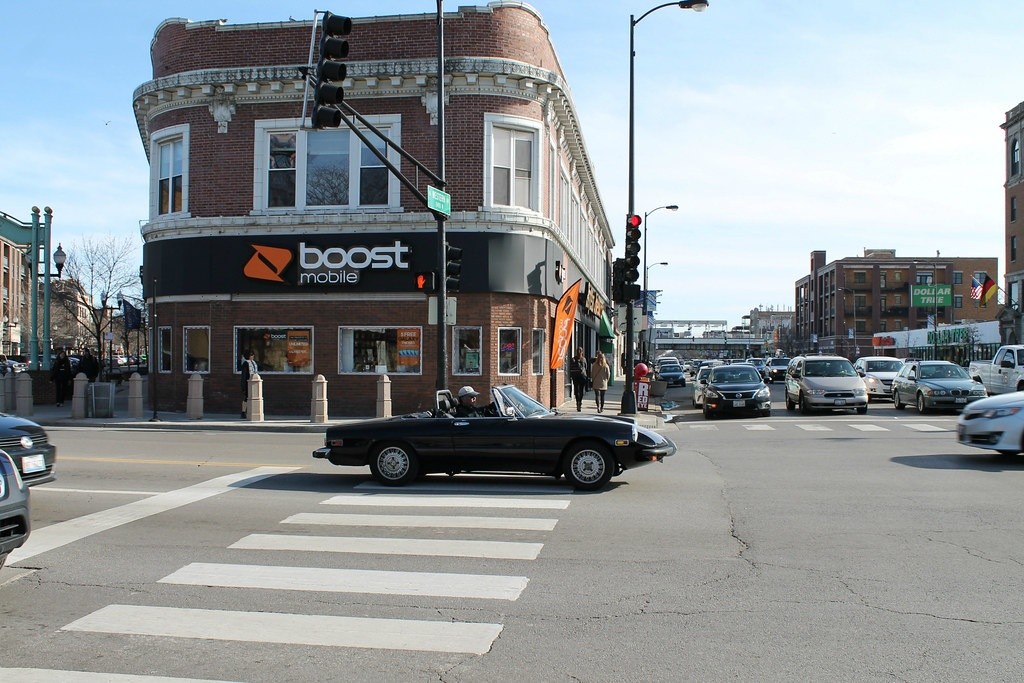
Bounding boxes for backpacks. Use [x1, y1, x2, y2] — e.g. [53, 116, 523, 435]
[570, 359, 583, 376]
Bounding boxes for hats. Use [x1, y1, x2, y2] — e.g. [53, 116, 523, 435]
[458, 386, 480, 397]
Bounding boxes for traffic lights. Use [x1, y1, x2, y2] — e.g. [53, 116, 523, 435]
[445, 241, 463, 293]
[413, 271, 436, 292]
[312, 10, 354, 129]
[624, 214, 642, 282]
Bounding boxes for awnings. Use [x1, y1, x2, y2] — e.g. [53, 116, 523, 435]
[600, 310, 615, 340]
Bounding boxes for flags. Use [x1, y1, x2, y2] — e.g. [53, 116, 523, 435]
[970, 277, 983, 300]
[979, 275, 999, 305]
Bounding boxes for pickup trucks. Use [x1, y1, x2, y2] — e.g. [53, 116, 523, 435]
[968, 344, 1024, 398]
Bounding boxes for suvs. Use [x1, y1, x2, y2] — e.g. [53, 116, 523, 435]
[784, 353, 868, 415]
[766, 357, 791, 384]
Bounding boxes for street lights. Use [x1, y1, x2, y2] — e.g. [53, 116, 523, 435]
[620, 0, 710, 415]
[100, 289, 124, 373]
[643, 205, 679, 368]
[844, 287, 856, 364]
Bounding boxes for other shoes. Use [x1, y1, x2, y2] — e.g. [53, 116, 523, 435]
[241, 412, 247, 418]
[57, 402, 60, 407]
[577, 402, 581, 412]
[598, 408, 604, 412]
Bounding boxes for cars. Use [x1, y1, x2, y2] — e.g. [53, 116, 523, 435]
[892, 360, 989, 415]
[956, 390, 1024, 456]
[690, 366, 712, 409]
[0, 413, 56, 488]
[0, 449, 31, 571]
[656, 356, 767, 387]
[0, 353, 148, 375]
[853, 356, 915, 403]
[700, 365, 773, 419]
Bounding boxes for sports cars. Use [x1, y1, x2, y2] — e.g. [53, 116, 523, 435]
[312, 382, 677, 492]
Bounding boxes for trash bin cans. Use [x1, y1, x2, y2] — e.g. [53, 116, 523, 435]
[90, 382, 115, 418]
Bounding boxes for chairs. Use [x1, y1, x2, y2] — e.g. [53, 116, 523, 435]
[434, 390, 459, 418]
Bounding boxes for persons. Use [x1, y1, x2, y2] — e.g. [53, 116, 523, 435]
[240, 350, 257, 418]
[591, 351, 610, 414]
[50, 351, 71, 407]
[457, 385, 496, 417]
[570, 347, 587, 411]
[78, 349, 99, 383]
